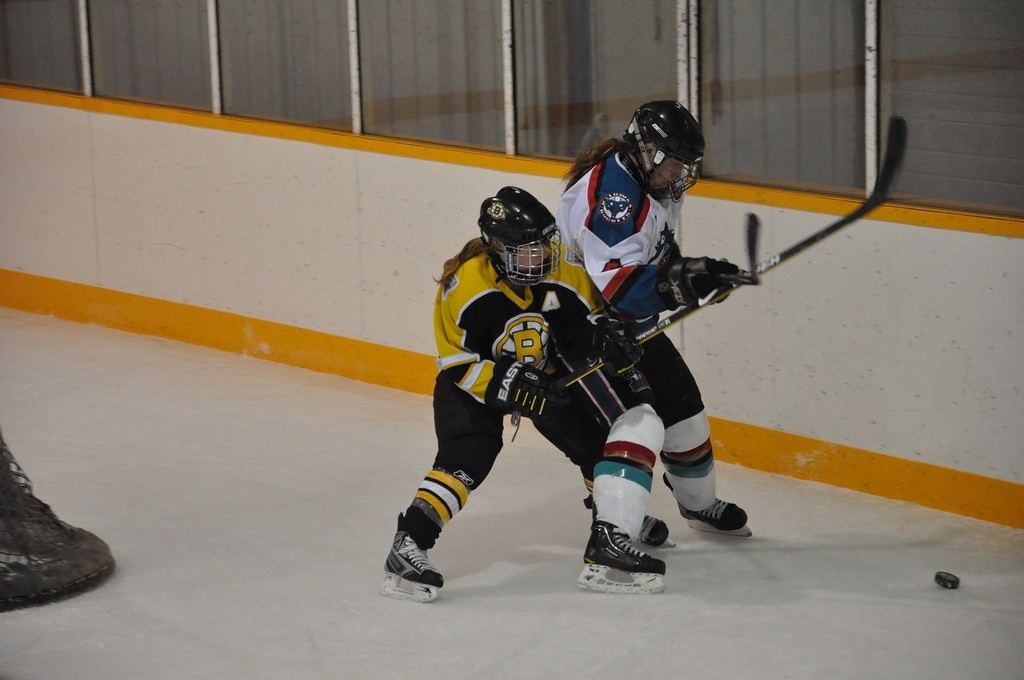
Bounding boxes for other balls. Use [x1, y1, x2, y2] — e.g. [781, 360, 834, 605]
[934, 571, 959, 588]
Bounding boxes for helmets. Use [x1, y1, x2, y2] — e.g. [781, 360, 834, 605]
[623, 100, 706, 201]
[477, 186, 560, 288]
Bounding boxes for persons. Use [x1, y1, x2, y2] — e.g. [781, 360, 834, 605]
[382, 98, 753, 603]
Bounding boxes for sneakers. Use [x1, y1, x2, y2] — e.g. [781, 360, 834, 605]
[576, 521, 666, 594]
[663, 474, 752, 538]
[378, 512, 443, 602]
[584, 494, 677, 548]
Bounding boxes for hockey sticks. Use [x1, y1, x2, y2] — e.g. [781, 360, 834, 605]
[555, 115, 909, 388]
[721, 213, 762, 286]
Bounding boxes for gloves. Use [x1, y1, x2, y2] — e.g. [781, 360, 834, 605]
[559, 312, 633, 358]
[668, 257, 739, 310]
[490, 355, 576, 420]
